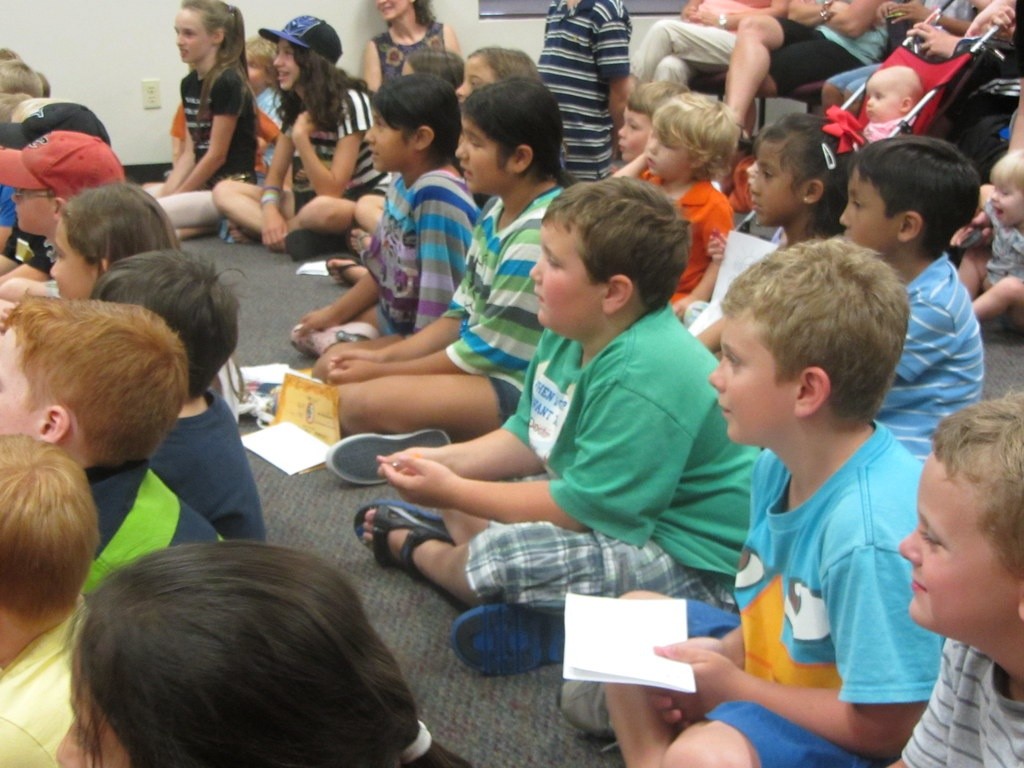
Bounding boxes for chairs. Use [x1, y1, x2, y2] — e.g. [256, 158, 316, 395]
[715, 69, 839, 134]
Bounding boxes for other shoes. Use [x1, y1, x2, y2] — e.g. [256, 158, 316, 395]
[555, 678, 618, 752]
[739, 130, 754, 150]
[284, 229, 350, 261]
[450, 601, 565, 677]
[326, 430, 452, 485]
[226, 219, 261, 244]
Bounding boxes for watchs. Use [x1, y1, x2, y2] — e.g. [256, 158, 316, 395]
[718, 9, 727, 29]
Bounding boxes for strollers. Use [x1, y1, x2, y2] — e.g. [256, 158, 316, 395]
[732, 0, 1003, 248]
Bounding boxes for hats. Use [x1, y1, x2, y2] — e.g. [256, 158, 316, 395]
[0, 131, 126, 199]
[1, 102, 110, 149]
[258, 15, 343, 65]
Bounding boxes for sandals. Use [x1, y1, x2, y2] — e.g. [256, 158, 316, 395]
[353, 500, 454, 588]
[326, 256, 358, 285]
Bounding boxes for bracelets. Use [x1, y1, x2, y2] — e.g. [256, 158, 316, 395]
[262, 194, 279, 206]
[263, 185, 281, 192]
[820, 1, 831, 22]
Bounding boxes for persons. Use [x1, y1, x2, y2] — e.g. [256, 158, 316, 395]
[2, 1, 1023, 768]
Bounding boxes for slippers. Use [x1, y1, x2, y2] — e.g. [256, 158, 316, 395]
[324, 321, 381, 341]
[290, 323, 360, 360]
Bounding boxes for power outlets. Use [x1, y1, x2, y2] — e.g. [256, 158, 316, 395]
[142, 78, 162, 110]
